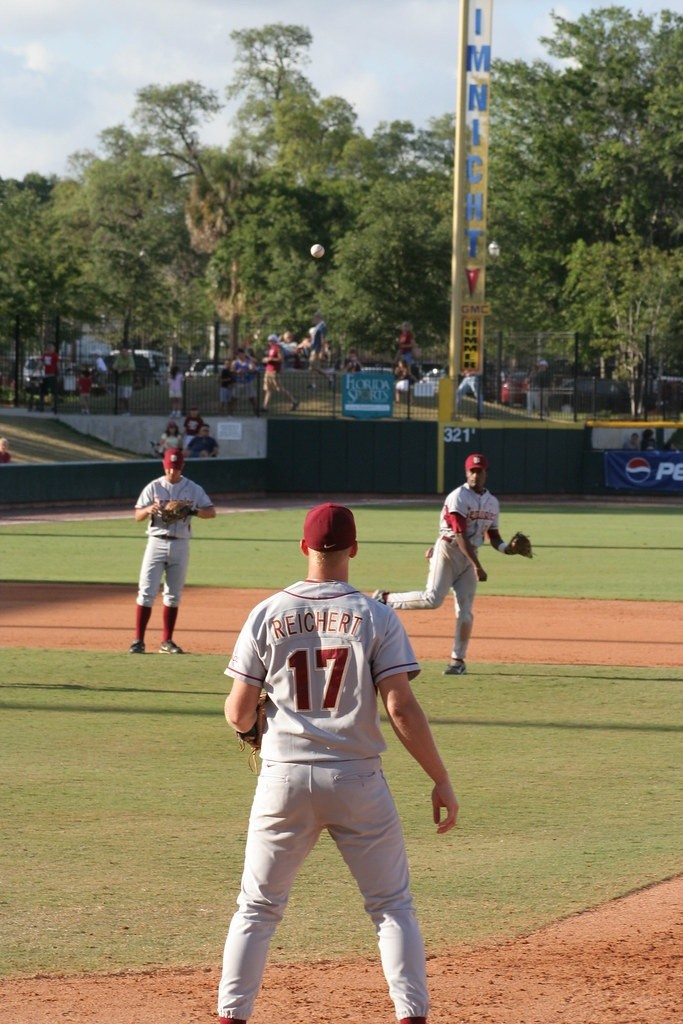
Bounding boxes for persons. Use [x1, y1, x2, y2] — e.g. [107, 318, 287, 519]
[219, 330, 299, 415]
[130, 448, 215, 654]
[77, 347, 134, 420]
[510, 358, 555, 417]
[392, 321, 424, 404]
[456, 375, 485, 418]
[224, 503, 459, 1023]
[625, 429, 679, 452]
[34, 342, 59, 413]
[169, 366, 185, 418]
[298, 313, 362, 390]
[0, 436, 11, 464]
[371, 451, 522, 675]
[159, 405, 220, 460]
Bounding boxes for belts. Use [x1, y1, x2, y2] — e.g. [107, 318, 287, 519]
[443, 536, 453, 544]
[156, 534, 183, 543]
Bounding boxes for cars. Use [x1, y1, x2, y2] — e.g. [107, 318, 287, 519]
[19, 345, 228, 395]
[425, 360, 683, 417]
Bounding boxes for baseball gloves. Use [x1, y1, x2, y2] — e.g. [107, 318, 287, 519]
[506, 534, 533, 558]
[236, 692, 266, 753]
[161, 501, 193, 524]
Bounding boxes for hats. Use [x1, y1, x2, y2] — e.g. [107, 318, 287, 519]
[465, 454, 488, 472]
[268, 334, 279, 342]
[164, 449, 184, 472]
[304, 503, 357, 552]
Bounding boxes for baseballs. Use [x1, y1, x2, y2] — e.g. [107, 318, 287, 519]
[310, 243, 325, 258]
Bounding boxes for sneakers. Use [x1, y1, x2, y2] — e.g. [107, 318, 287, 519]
[371, 589, 389, 604]
[159, 640, 182, 653]
[130, 640, 145, 654]
[442, 662, 467, 674]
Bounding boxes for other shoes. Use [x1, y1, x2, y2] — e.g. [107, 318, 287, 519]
[260, 407, 267, 412]
[170, 410, 181, 418]
[293, 401, 300, 411]
